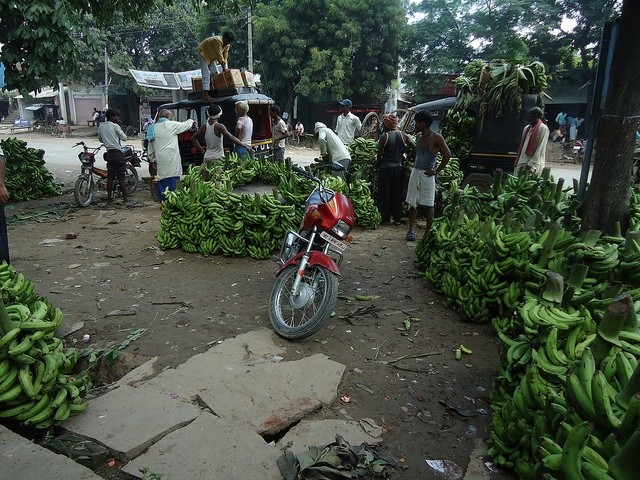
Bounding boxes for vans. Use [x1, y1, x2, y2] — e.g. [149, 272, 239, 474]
[395, 94, 541, 194]
[142, 86, 275, 175]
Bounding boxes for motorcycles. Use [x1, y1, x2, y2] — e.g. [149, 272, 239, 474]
[269, 164, 354, 340]
[572, 138, 586, 166]
[71, 141, 142, 208]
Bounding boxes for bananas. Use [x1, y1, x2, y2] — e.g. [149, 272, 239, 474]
[441, 57, 550, 136]
[306, 136, 465, 207]
[413, 170, 640, 480]
[0, 258, 94, 432]
[0, 136, 64, 203]
[156, 151, 382, 259]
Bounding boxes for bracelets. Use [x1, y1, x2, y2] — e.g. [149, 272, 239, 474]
[243, 144, 247, 148]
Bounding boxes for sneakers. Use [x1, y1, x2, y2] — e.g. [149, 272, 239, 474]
[406, 231, 416, 240]
[394, 217, 401, 224]
[382, 216, 390, 225]
[203, 95, 213, 100]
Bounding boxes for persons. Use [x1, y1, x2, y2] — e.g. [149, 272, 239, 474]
[405, 110, 452, 241]
[98, 109, 128, 205]
[196, 31, 234, 101]
[314, 121, 351, 179]
[291, 120, 304, 145]
[148, 109, 195, 204]
[513, 106, 550, 177]
[363, 111, 382, 141]
[233, 100, 253, 162]
[377, 113, 416, 226]
[551, 111, 585, 149]
[282, 111, 293, 144]
[269, 105, 289, 163]
[334, 98, 362, 146]
[0, 146, 10, 265]
[191, 105, 255, 189]
[91, 107, 106, 127]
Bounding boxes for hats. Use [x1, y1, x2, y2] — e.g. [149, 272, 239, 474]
[339, 98, 352, 109]
[313, 121, 327, 139]
[383, 114, 398, 130]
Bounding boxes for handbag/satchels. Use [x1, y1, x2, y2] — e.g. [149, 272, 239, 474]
[107, 149, 126, 165]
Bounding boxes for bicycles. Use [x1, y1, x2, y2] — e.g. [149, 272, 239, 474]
[287, 123, 314, 148]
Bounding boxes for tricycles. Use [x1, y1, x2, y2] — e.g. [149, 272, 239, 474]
[45, 123, 73, 138]
